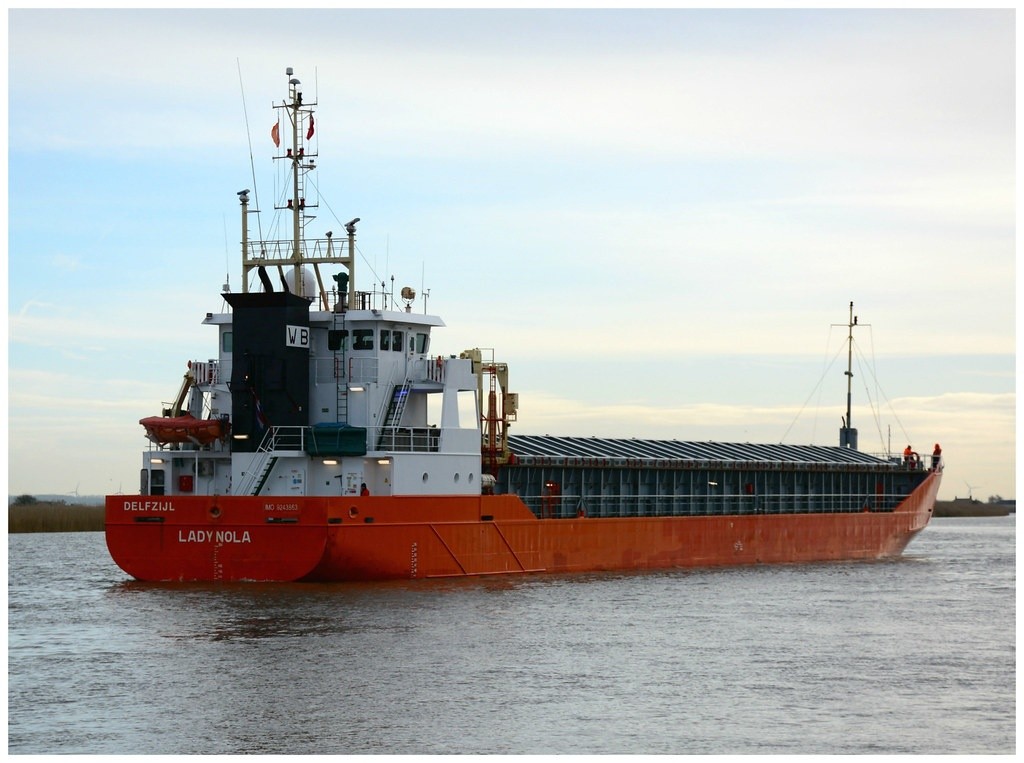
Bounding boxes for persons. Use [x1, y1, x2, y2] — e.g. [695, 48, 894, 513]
[361, 483, 369, 495]
[931, 444, 941, 471]
[904, 445, 912, 461]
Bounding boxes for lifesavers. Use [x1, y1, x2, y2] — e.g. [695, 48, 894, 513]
[906, 451, 921, 465]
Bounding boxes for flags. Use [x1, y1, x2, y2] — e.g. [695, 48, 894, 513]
[271, 123, 280, 147]
[307, 115, 314, 140]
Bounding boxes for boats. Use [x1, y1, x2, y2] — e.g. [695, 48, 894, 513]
[105, 58, 945, 585]
